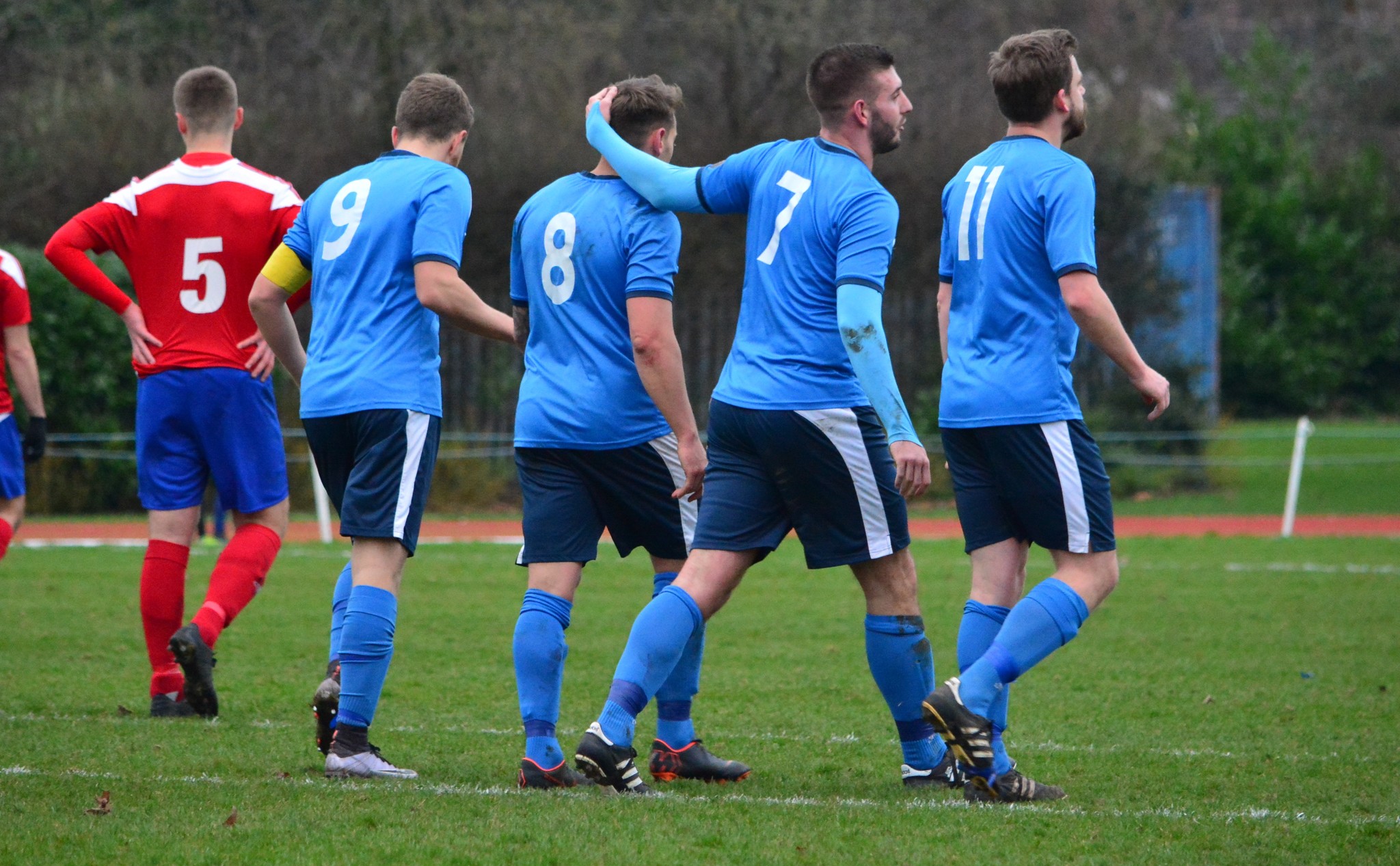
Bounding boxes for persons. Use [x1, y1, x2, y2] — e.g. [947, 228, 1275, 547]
[509, 75, 753, 786]
[247, 71, 514, 777]
[923, 29, 1170, 805]
[574, 45, 971, 794]
[43, 64, 312, 716]
[0, 248, 47, 561]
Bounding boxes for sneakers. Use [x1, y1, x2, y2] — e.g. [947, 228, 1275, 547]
[575, 722, 663, 797]
[311, 665, 341, 757]
[168, 621, 218, 720]
[150, 693, 197, 718]
[517, 758, 595, 790]
[964, 769, 1067, 804]
[922, 676, 993, 779]
[325, 730, 418, 779]
[650, 737, 751, 785]
[901, 743, 1017, 790]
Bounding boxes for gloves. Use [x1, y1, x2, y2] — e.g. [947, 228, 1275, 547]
[21, 416, 47, 461]
